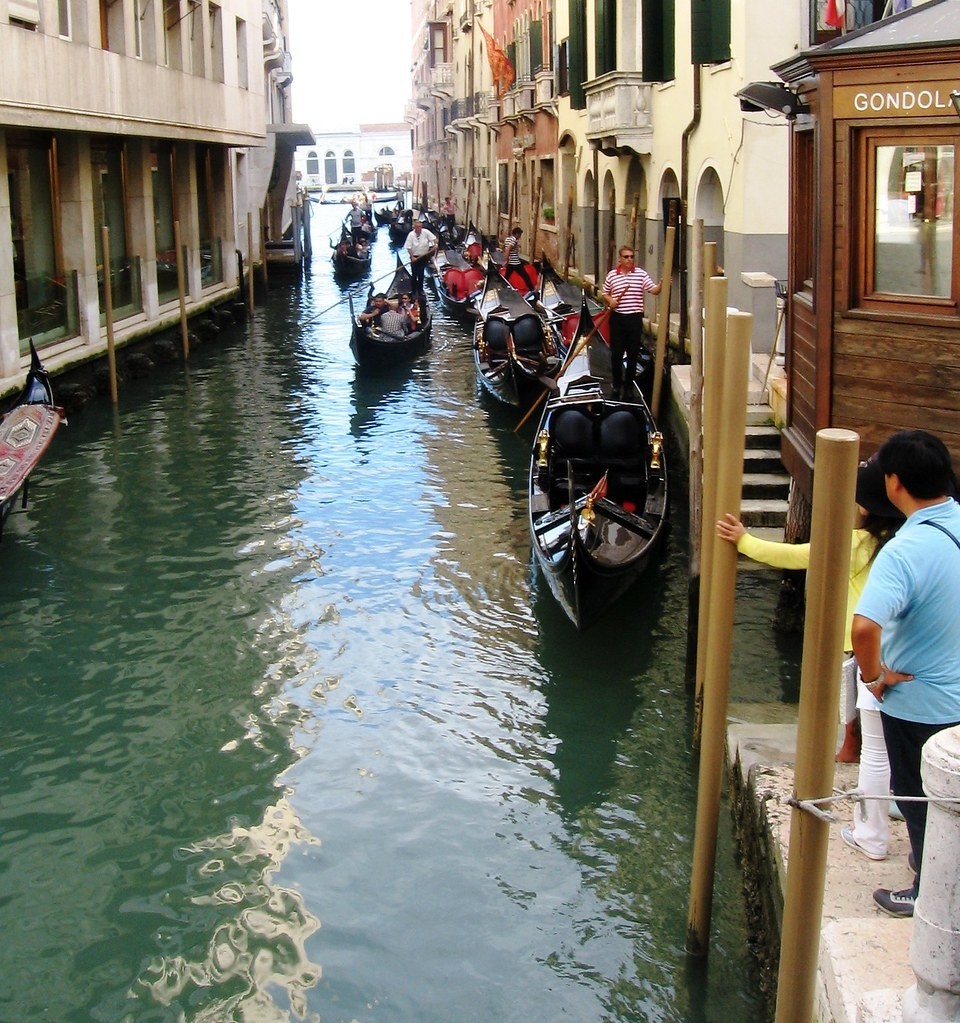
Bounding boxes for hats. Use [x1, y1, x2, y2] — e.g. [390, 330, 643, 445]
[856, 454, 901, 513]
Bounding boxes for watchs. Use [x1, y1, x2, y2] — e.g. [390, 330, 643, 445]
[859, 667, 886, 691]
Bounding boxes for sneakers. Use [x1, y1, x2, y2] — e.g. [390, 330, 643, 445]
[873, 888, 918, 918]
[908, 853, 918, 874]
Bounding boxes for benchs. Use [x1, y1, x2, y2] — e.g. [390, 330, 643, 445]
[501, 264, 537, 292]
[563, 308, 610, 346]
[468, 243, 482, 258]
[443, 268, 482, 297]
[549, 405, 652, 466]
[484, 313, 544, 354]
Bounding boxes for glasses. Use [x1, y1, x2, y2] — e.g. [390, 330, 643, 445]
[621, 254, 634, 259]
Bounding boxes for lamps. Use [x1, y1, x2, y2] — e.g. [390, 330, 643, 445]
[734, 80, 810, 125]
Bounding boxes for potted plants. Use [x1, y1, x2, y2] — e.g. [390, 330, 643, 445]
[544, 208, 554, 225]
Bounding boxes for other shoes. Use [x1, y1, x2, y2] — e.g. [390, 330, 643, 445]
[612, 386, 635, 400]
[887, 799, 906, 822]
[841, 826, 886, 860]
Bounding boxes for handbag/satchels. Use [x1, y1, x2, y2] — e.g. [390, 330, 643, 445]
[839, 656, 858, 724]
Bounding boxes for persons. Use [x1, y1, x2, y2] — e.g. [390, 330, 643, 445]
[601, 246, 674, 403]
[333, 176, 533, 338]
[718, 453, 908, 861]
[850, 428, 959, 919]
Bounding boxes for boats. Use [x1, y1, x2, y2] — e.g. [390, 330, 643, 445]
[0, 337, 63, 528]
[390, 206, 605, 409]
[334, 199, 405, 277]
[308, 192, 404, 206]
[528, 285, 668, 633]
[349, 251, 433, 375]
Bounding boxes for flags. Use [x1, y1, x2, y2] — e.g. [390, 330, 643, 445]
[480, 30, 515, 100]
